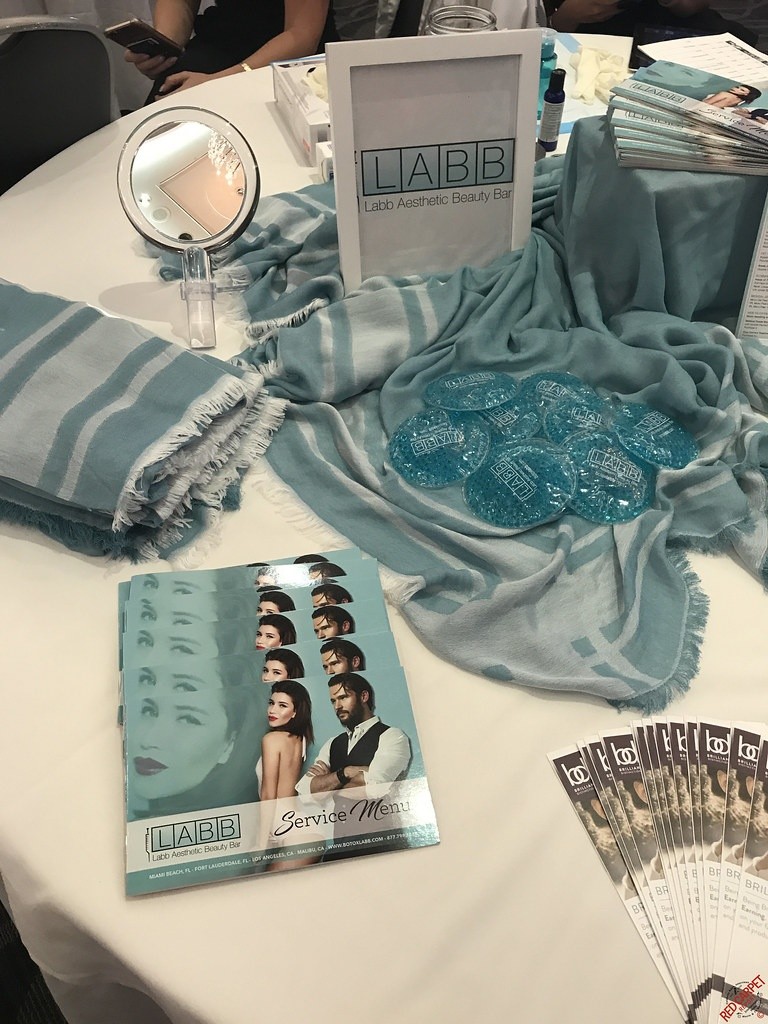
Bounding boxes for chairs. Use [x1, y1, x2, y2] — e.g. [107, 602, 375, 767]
[0, 16, 122, 198]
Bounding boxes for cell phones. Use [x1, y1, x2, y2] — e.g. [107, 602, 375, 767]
[104, 17, 185, 60]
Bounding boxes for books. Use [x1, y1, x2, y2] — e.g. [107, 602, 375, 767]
[116, 546, 440, 896]
[547, 714, 768, 1024]
[607, 60, 768, 176]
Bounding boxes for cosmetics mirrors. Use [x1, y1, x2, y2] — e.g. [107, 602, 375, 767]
[116, 105, 261, 349]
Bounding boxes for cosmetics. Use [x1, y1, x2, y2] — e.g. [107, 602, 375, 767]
[536, 43, 559, 118]
[538, 68, 569, 151]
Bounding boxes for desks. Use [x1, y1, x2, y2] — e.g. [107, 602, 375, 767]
[0, 66, 768, 1024]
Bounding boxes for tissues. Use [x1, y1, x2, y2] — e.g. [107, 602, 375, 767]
[268, 56, 330, 167]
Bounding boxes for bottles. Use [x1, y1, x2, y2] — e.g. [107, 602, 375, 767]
[423, 6, 497, 36]
[538, 68, 566, 152]
[537, 27, 558, 120]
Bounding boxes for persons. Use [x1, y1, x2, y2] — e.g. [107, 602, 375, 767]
[133, 555, 413, 872]
[125, 0, 340, 107]
[634, 60, 768, 126]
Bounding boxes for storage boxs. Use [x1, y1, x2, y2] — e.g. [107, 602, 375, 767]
[270, 54, 332, 167]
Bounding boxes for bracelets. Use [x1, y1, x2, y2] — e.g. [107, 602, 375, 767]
[240, 62, 252, 72]
